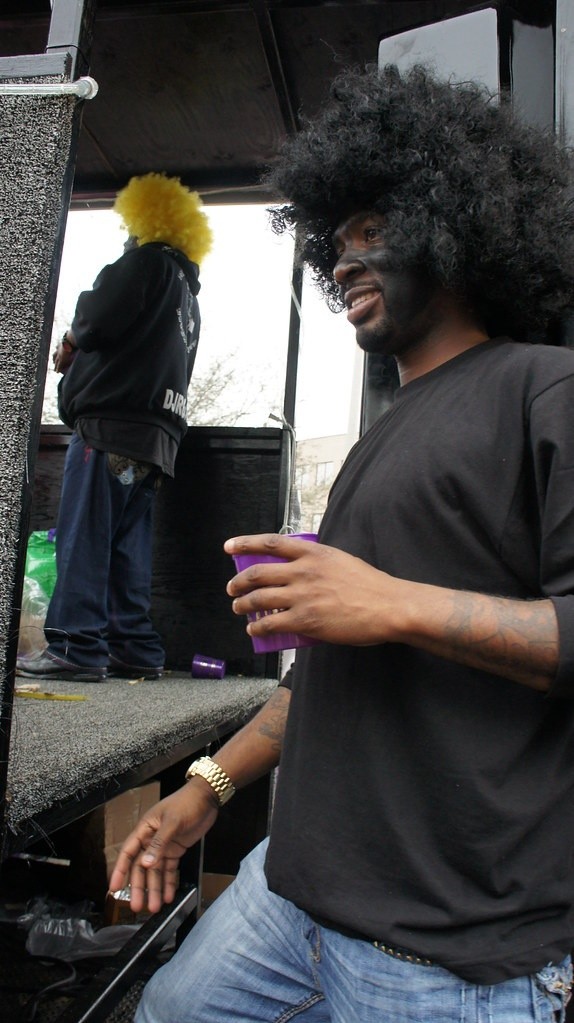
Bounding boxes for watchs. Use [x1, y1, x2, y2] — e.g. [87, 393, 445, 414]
[62, 331, 76, 353]
[185, 755, 236, 806]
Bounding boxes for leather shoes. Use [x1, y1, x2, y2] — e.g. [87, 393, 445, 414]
[16, 654, 105, 683]
[108, 650, 164, 679]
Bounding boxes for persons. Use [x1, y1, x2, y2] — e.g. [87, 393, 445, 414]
[106, 62, 574, 1023]
[15, 170, 214, 683]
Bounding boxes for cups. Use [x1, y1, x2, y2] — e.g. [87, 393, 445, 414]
[231, 532, 320, 653]
[191, 653, 226, 678]
